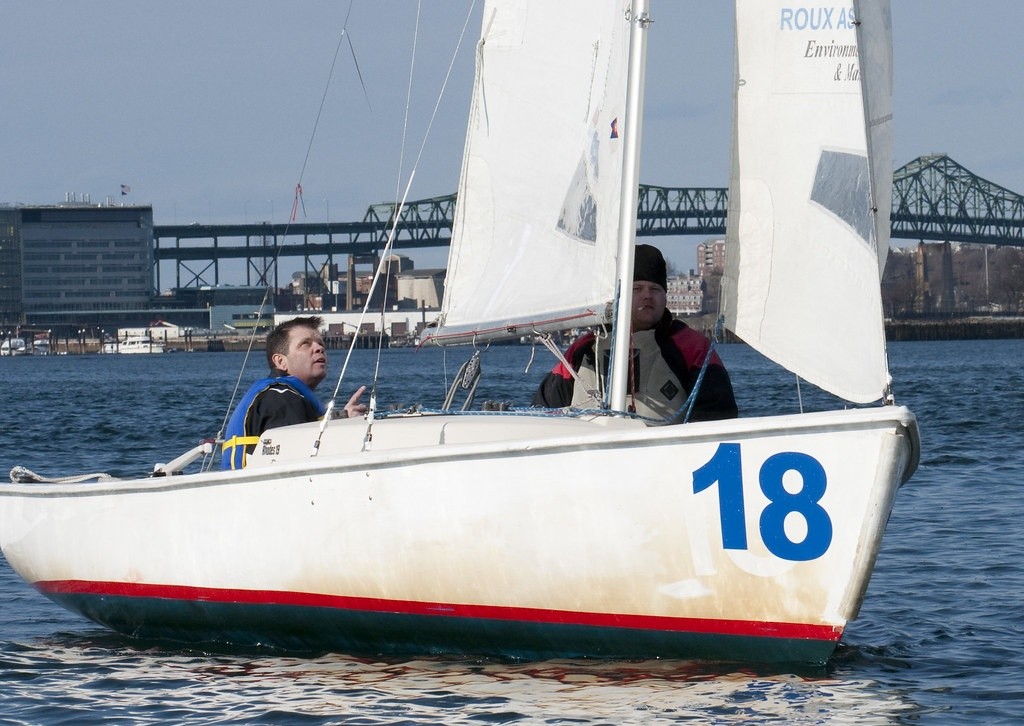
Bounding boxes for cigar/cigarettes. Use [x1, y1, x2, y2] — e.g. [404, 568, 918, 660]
[638, 306, 643, 310]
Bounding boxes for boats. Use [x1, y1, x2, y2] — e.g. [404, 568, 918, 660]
[97, 336, 164, 354]
[0, 338, 51, 356]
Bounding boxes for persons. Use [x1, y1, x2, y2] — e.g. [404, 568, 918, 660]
[221, 317, 367, 470]
[530, 243, 737, 426]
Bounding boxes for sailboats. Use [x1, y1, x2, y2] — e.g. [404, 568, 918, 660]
[0, 0, 923, 679]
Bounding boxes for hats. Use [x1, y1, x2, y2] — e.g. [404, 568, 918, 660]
[633, 245, 667, 294]
